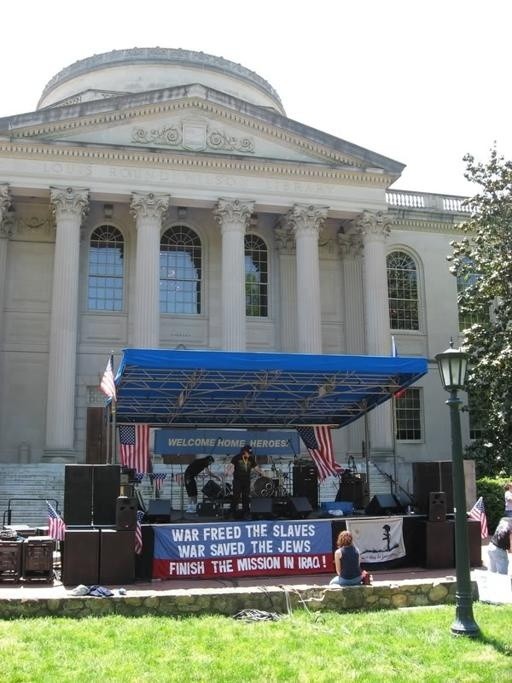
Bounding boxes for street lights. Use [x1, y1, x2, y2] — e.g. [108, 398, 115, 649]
[434, 335, 469, 637]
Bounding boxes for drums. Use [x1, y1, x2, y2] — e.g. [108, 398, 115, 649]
[270, 478, 279, 490]
[200, 480, 220, 496]
[250, 476, 275, 497]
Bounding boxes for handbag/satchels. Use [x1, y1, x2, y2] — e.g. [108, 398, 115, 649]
[361, 569, 371, 585]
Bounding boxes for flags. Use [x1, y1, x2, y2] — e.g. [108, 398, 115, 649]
[97, 353, 118, 402]
[119, 424, 153, 481]
[47, 502, 66, 542]
[470, 496, 489, 539]
[131, 471, 185, 491]
[134, 510, 145, 555]
[296, 426, 344, 480]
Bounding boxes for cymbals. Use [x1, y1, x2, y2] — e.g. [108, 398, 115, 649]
[272, 457, 291, 461]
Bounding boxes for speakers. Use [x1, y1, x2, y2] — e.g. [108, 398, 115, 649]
[64, 464, 93, 525]
[285, 496, 313, 519]
[440, 462, 455, 513]
[447, 518, 483, 567]
[250, 497, 273, 520]
[116, 496, 137, 531]
[149, 499, 171, 524]
[412, 462, 440, 514]
[93, 464, 121, 525]
[63, 526, 99, 586]
[428, 492, 446, 522]
[365, 494, 398, 515]
[100, 527, 135, 586]
[416, 518, 454, 569]
[392, 493, 412, 515]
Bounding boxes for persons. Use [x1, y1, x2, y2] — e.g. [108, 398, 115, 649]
[225, 445, 268, 521]
[487, 484, 512, 576]
[328, 530, 363, 585]
[185, 456, 223, 513]
